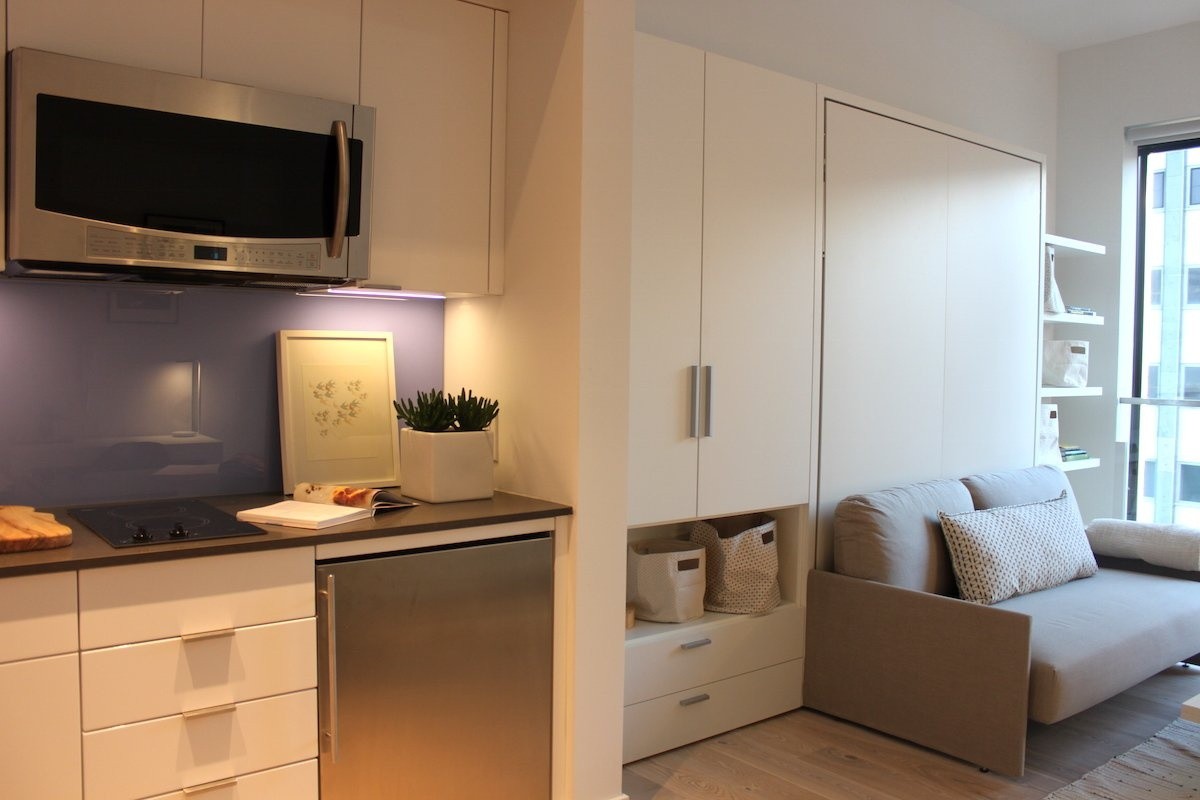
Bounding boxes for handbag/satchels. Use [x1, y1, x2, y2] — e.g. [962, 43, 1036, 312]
[689, 512, 782, 614]
[1035, 403, 1062, 467]
[625, 539, 707, 623]
[1041, 340, 1090, 388]
[1043, 245, 1067, 314]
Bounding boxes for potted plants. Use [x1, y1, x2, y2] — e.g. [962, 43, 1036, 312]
[393, 387, 500, 503]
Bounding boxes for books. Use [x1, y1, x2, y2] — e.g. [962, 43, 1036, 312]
[1059, 447, 1088, 461]
[1065, 306, 1096, 315]
[236, 482, 420, 529]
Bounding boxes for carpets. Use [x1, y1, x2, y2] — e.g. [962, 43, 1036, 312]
[1043, 716, 1200, 800]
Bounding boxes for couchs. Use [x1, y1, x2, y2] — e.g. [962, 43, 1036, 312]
[806, 465, 1200, 779]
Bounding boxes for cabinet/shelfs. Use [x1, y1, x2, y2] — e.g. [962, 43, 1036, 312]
[0, 517, 567, 800]
[1043, 230, 1108, 471]
[624, 594, 802, 765]
[605, 23, 816, 525]
[7, 0, 510, 297]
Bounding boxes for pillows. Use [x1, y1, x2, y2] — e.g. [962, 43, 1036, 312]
[938, 488, 1099, 605]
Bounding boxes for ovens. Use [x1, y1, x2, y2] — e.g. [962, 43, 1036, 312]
[3, 46, 380, 289]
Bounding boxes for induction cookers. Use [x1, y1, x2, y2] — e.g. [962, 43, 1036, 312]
[67, 501, 270, 549]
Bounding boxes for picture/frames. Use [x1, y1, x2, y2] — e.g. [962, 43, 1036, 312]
[276, 330, 401, 494]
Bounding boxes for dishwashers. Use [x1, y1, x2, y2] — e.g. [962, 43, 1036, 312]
[315, 534, 553, 799]
